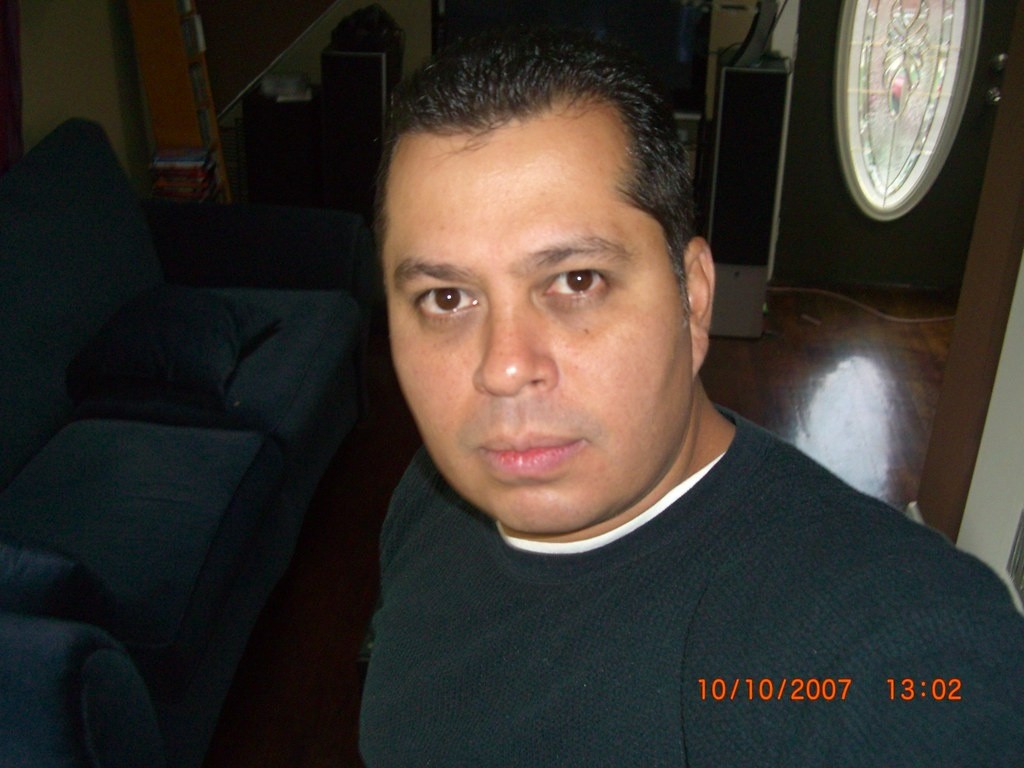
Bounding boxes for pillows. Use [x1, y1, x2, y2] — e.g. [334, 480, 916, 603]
[0, 529, 169, 647]
[66, 289, 285, 413]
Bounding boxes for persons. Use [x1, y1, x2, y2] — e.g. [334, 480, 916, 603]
[358, 23, 1024, 768]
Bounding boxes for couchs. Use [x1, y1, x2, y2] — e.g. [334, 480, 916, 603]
[0, 116, 386, 768]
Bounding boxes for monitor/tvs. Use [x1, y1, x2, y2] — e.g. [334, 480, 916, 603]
[432, 0, 713, 120]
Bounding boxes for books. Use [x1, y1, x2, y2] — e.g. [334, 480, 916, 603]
[151, 146, 218, 200]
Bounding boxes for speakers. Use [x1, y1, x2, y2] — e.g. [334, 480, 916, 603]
[321, 30, 403, 228]
[695, 66, 792, 281]
